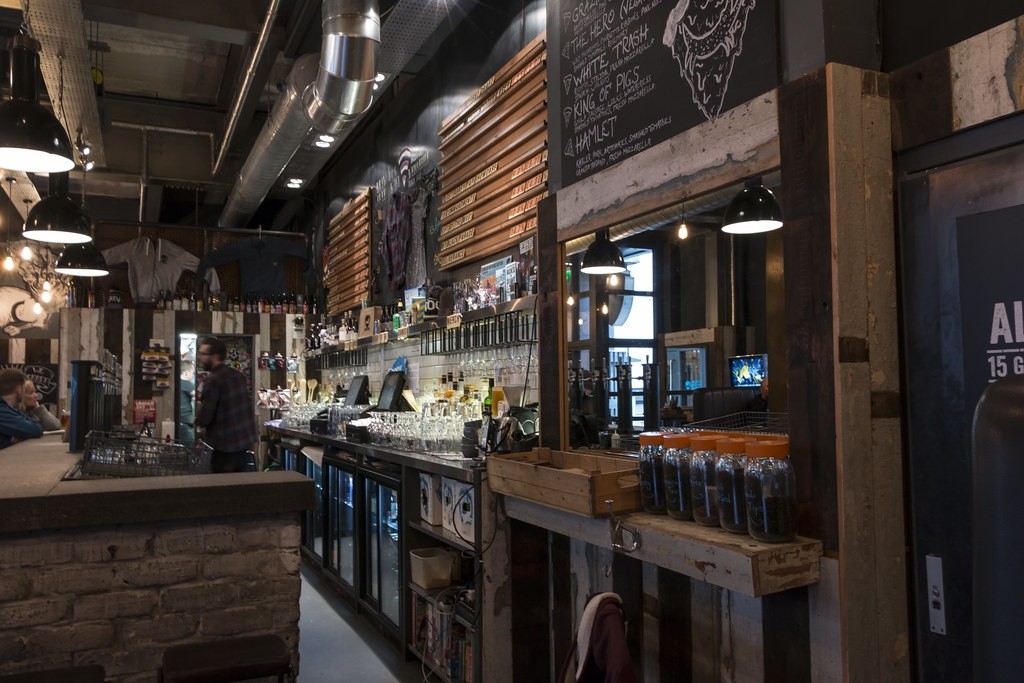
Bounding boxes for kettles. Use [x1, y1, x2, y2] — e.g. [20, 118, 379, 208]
[496, 411, 524, 454]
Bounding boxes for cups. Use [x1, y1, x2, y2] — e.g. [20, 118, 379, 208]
[399, 311, 412, 327]
[365, 410, 483, 458]
[61, 411, 70, 429]
[412, 298, 426, 324]
[259, 388, 371, 440]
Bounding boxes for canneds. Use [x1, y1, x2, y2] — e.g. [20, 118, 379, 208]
[393, 313, 399, 329]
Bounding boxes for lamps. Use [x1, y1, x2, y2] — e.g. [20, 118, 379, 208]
[73, 140, 90, 155]
[0, 27, 77, 177]
[21, 168, 94, 245]
[581, 226, 627, 275]
[722, 176, 782, 234]
[78, 158, 95, 171]
[53, 243, 109, 277]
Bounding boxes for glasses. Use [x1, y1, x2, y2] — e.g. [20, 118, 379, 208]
[197, 352, 212, 357]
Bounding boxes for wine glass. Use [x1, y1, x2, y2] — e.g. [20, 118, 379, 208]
[442, 338, 539, 377]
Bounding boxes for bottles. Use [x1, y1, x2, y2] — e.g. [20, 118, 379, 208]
[433, 370, 505, 420]
[155, 287, 303, 315]
[638, 430, 797, 542]
[599, 429, 621, 449]
[303, 295, 403, 349]
[68, 276, 124, 308]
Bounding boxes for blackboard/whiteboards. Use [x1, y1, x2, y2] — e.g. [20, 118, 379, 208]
[546, 0, 781, 195]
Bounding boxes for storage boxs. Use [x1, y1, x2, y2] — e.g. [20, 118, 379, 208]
[439, 475, 457, 536]
[453, 482, 478, 543]
[419, 474, 452, 525]
[485, 447, 642, 518]
[407, 548, 452, 589]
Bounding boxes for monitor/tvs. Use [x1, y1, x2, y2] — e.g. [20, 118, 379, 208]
[727, 354, 768, 387]
[376, 371, 406, 412]
[344, 375, 368, 407]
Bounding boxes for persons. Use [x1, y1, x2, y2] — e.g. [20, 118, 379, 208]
[19, 380, 62, 432]
[750, 379, 768, 425]
[0, 369, 42, 450]
[191, 335, 256, 474]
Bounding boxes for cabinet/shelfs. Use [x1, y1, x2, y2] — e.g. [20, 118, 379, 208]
[405, 467, 477, 683]
